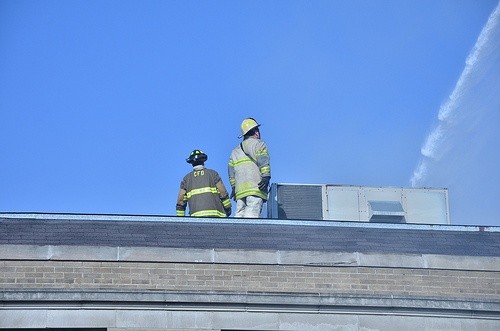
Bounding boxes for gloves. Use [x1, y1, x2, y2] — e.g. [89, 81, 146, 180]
[258, 176, 271, 192]
[230, 186, 237, 203]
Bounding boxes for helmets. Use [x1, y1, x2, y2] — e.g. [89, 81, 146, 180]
[186, 149, 209, 163]
[237, 118, 261, 138]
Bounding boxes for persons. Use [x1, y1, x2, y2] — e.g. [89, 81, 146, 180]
[227, 118, 271, 219]
[176, 150, 232, 218]
